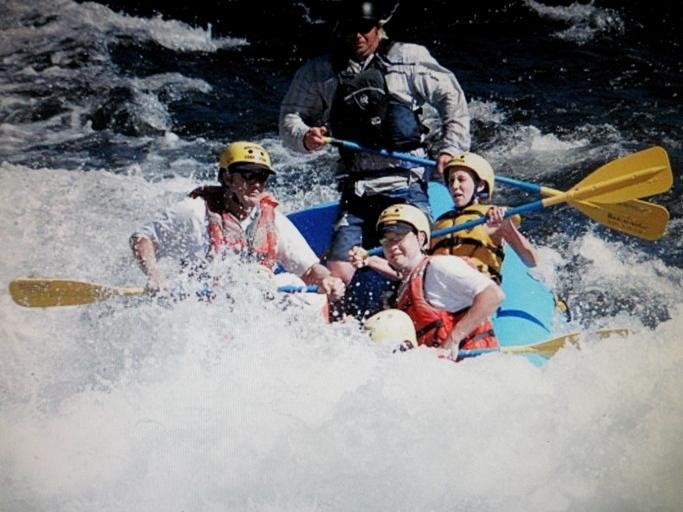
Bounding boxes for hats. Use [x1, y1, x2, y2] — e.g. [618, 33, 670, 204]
[373, 222, 414, 236]
[230, 161, 276, 178]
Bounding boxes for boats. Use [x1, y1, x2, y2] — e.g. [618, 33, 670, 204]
[283, 178, 567, 366]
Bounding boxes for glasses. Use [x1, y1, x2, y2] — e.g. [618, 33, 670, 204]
[378, 235, 405, 245]
[232, 170, 269, 180]
[342, 25, 373, 36]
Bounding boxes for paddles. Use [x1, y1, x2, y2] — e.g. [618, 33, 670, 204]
[322, 135, 669, 241]
[355, 145, 673, 260]
[437, 328, 630, 358]
[8, 279, 336, 309]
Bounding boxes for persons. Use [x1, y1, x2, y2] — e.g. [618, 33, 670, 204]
[129, 140, 346, 302]
[278, 0, 473, 286]
[377, 203, 504, 363]
[330, 152, 537, 321]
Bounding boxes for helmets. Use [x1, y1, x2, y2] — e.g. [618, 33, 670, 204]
[443, 154, 494, 202]
[364, 309, 417, 350]
[375, 204, 430, 250]
[333, 1, 379, 25]
[217, 141, 276, 182]
